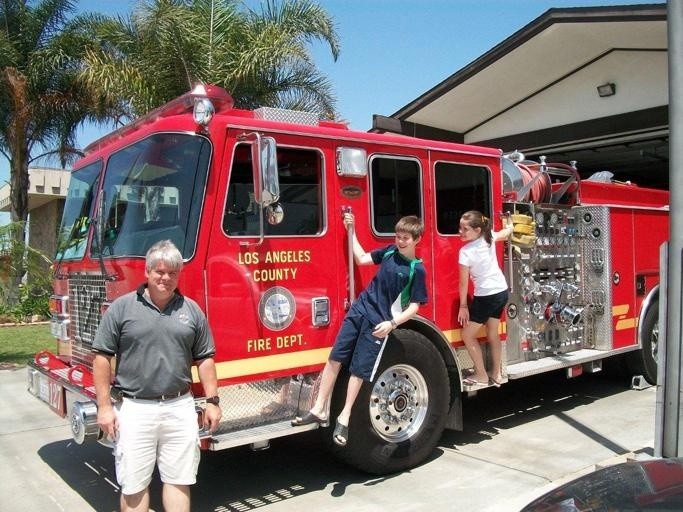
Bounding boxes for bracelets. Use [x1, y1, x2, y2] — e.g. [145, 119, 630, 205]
[459, 305, 464, 308]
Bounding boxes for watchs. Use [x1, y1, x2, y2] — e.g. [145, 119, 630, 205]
[206, 396, 220, 404]
[390, 319, 398, 330]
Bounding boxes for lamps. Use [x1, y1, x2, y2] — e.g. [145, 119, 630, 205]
[597, 82, 615, 97]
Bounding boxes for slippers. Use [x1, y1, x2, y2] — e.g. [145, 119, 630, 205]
[332, 416, 350, 447]
[291, 410, 328, 426]
[489, 375, 502, 388]
[462, 375, 489, 386]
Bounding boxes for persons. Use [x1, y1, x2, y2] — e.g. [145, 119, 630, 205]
[267, 206, 278, 225]
[260, 383, 289, 417]
[90, 239, 223, 512]
[456, 210, 515, 388]
[291, 212, 429, 448]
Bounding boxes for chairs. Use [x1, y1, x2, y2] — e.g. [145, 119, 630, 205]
[149, 204, 177, 228]
[114, 201, 144, 226]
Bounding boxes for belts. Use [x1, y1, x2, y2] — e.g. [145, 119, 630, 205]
[123, 386, 191, 400]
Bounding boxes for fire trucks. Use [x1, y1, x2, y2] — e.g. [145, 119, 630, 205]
[29, 84, 669, 476]
